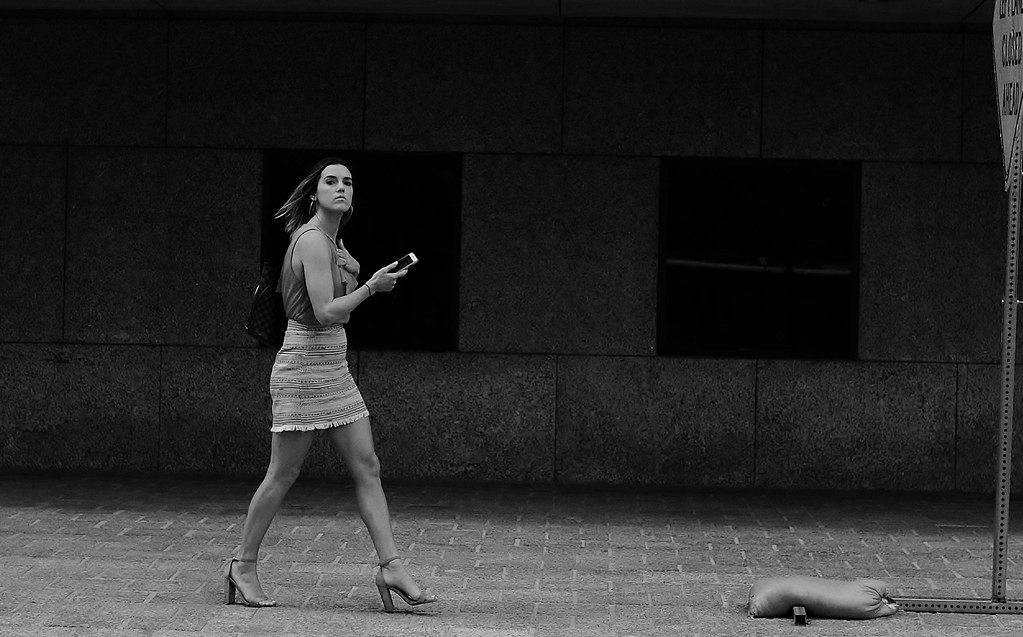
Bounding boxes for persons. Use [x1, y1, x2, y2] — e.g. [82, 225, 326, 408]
[224, 156, 439, 613]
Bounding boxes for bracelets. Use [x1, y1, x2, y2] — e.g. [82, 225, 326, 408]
[364, 284, 371, 296]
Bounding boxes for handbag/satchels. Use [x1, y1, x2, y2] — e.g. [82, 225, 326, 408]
[244, 262, 288, 350]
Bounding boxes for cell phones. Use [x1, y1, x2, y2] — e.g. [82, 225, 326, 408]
[387, 252, 419, 273]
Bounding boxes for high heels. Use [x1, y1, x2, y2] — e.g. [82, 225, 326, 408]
[225, 557, 277, 607]
[375, 556, 437, 612]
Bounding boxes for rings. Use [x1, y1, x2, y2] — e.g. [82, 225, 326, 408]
[394, 278, 396, 285]
[392, 286, 394, 289]
